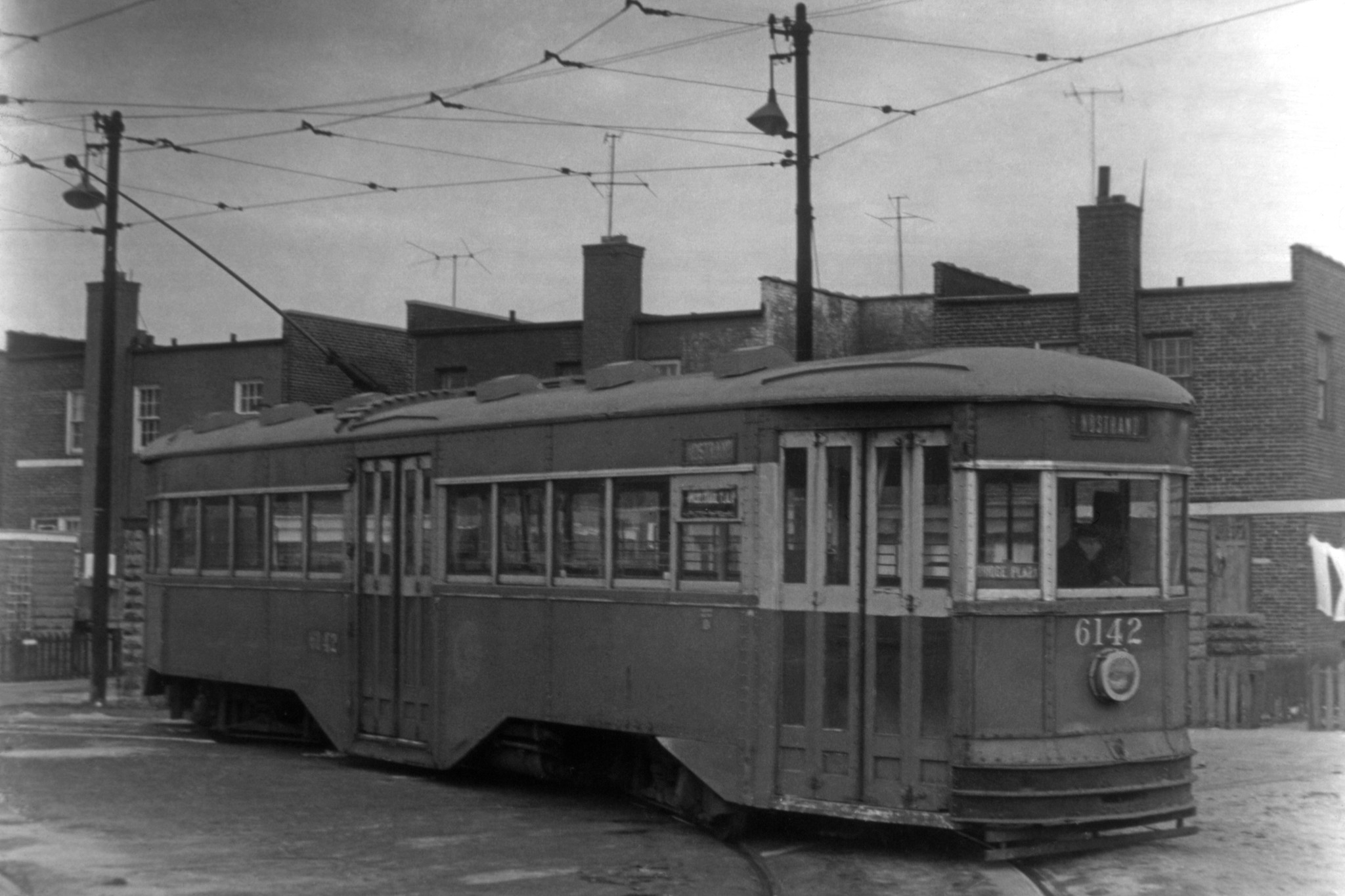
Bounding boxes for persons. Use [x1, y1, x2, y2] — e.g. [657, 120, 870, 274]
[1055, 520, 1126, 591]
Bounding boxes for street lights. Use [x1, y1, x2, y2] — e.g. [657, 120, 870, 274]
[746, 3, 814, 365]
[62, 109, 129, 707]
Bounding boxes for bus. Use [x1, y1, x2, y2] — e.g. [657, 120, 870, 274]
[143, 344, 1203, 854]
